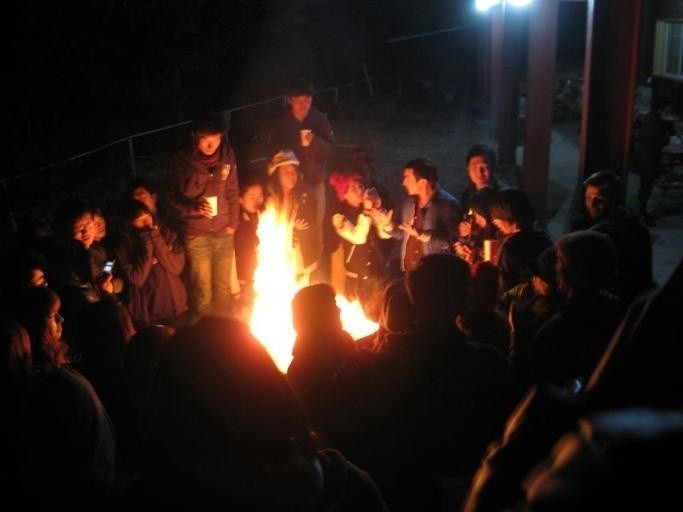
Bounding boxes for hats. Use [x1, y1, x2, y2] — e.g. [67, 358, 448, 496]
[266, 146, 301, 177]
[327, 170, 368, 205]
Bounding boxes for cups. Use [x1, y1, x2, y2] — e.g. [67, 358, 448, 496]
[483, 238, 499, 265]
[205, 195, 218, 217]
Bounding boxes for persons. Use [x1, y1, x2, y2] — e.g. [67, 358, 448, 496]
[628, 94, 678, 226]
[2, 144, 681, 508]
[167, 112, 240, 328]
[258, 81, 337, 267]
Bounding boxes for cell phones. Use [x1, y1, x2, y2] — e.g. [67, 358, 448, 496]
[101, 257, 116, 277]
[367, 187, 379, 203]
